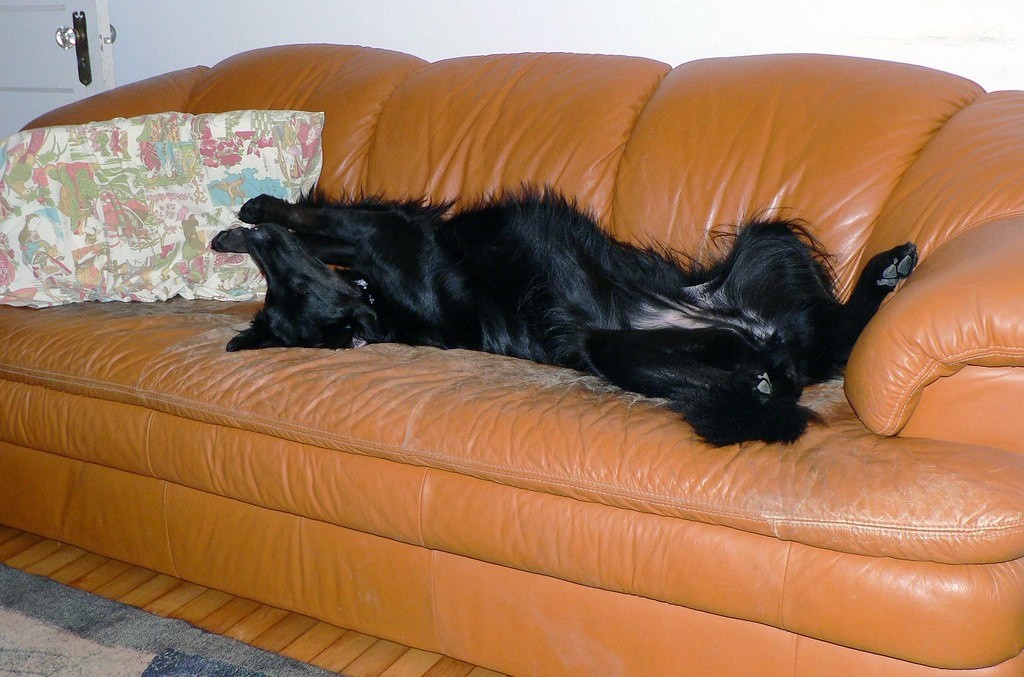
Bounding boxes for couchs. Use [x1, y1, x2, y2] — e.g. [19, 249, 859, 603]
[0, 42, 1024, 677]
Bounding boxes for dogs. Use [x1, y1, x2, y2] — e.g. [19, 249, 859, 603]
[211, 175, 922, 447]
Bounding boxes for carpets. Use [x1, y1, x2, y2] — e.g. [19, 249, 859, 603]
[0, 563, 346, 677]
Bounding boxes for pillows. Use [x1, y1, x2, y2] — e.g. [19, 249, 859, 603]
[0, 109, 325, 309]
